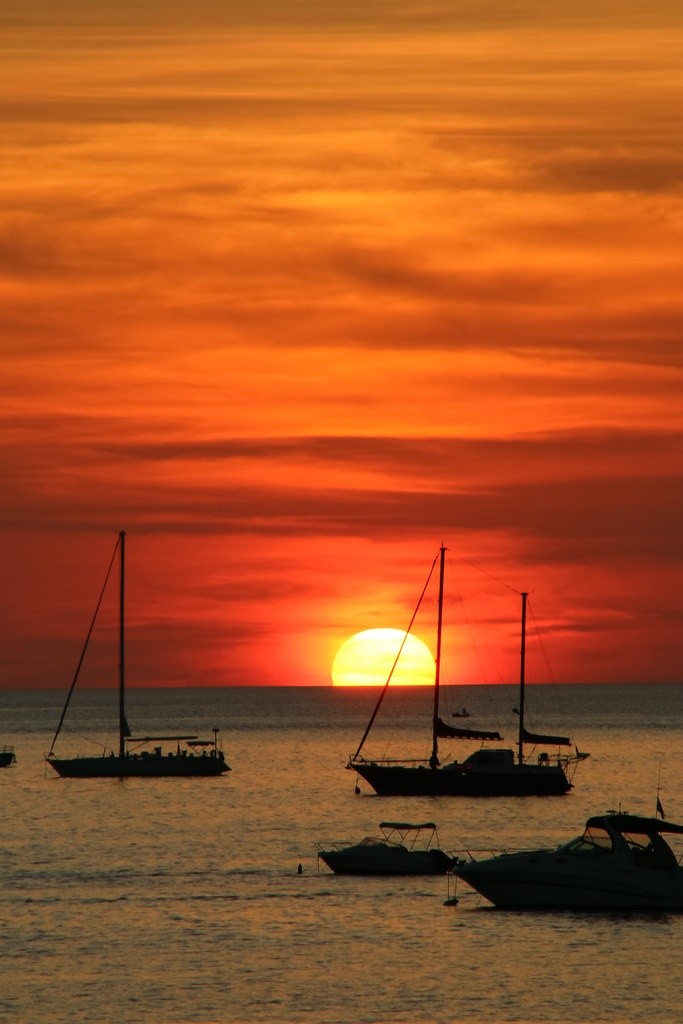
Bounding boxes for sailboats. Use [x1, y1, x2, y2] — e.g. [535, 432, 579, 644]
[44, 528, 234, 776]
[345, 539, 591, 797]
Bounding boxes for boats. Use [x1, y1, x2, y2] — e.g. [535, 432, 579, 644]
[449, 707, 470, 718]
[0, 742, 17, 767]
[314, 822, 457, 877]
[445, 763, 682, 917]
